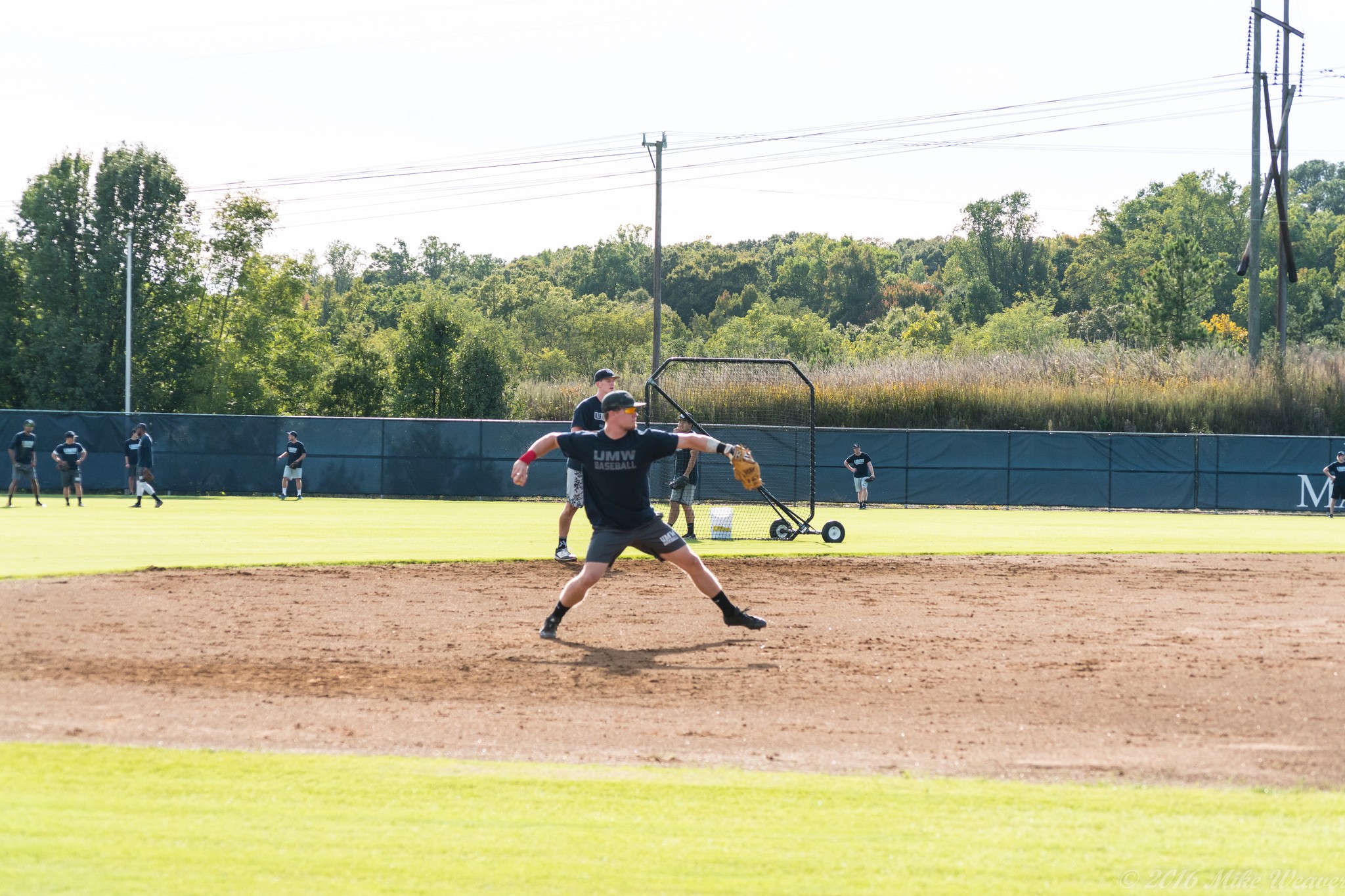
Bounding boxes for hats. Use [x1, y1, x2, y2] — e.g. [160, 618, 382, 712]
[65, 431, 79, 438]
[853, 444, 860, 447]
[601, 390, 647, 413]
[594, 368, 620, 383]
[23, 419, 36, 426]
[1337, 451, 1345, 456]
[675, 412, 694, 422]
[287, 431, 297, 437]
[133, 423, 146, 429]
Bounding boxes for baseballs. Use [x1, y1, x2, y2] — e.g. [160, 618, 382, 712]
[513, 473, 524, 485]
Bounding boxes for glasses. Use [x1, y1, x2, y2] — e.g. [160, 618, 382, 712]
[618, 407, 636, 415]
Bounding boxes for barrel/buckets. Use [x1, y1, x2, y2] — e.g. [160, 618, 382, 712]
[709, 508, 733, 538]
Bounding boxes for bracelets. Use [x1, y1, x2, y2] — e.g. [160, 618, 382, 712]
[519, 450, 537, 465]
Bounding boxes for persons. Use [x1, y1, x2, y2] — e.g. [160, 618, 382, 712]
[1323, 451, 1345, 518]
[554, 369, 620, 561]
[278, 431, 306, 499]
[844, 444, 875, 509]
[667, 413, 699, 539]
[129, 423, 163, 508]
[52, 432, 85, 506]
[511, 391, 767, 638]
[124, 429, 140, 495]
[7, 420, 42, 506]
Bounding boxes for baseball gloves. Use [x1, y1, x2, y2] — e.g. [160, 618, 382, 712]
[865, 476, 875, 482]
[730, 443, 762, 490]
[669, 475, 689, 489]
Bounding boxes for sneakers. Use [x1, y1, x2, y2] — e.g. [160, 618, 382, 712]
[859, 505, 863, 510]
[36, 501, 42, 506]
[66, 502, 70, 506]
[78, 502, 84, 507]
[278, 493, 286, 499]
[7, 502, 12, 507]
[862, 503, 867, 509]
[681, 533, 696, 539]
[539, 614, 561, 639]
[1329, 513, 1333, 518]
[298, 494, 302, 499]
[131, 503, 141, 508]
[723, 606, 766, 629]
[155, 499, 163, 508]
[554, 547, 577, 562]
